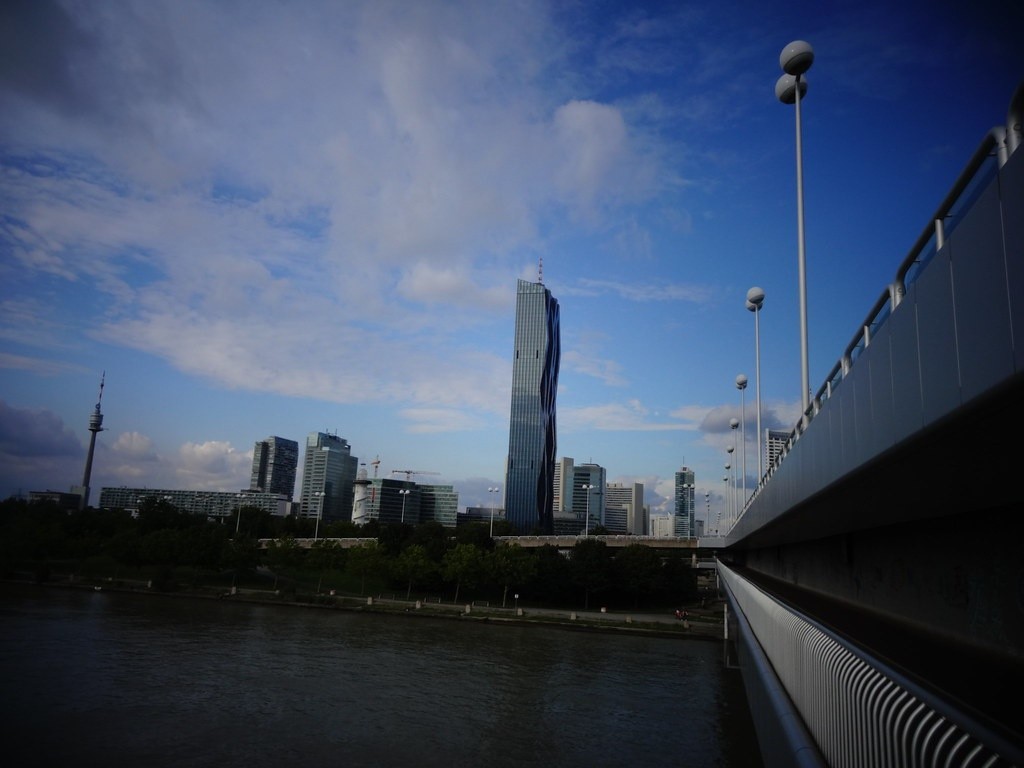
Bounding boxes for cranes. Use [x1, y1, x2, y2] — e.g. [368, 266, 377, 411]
[393, 470, 415, 481]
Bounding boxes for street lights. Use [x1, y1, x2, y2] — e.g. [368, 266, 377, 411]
[775, 42, 815, 429]
[745, 287, 765, 488]
[236, 494, 246, 532]
[736, 374, 750, 509]
[726, 445, 734, 529]
[582, 485, 593, 538]
[728, 418, 740, 521]
[684, 484, 695, 540]
[488, 487, 499, 538]
[315, 491, 326, 541]
[400, 490, 410, 522]
[706, 463, 731, 538]
[164, 496, 172, 503]
